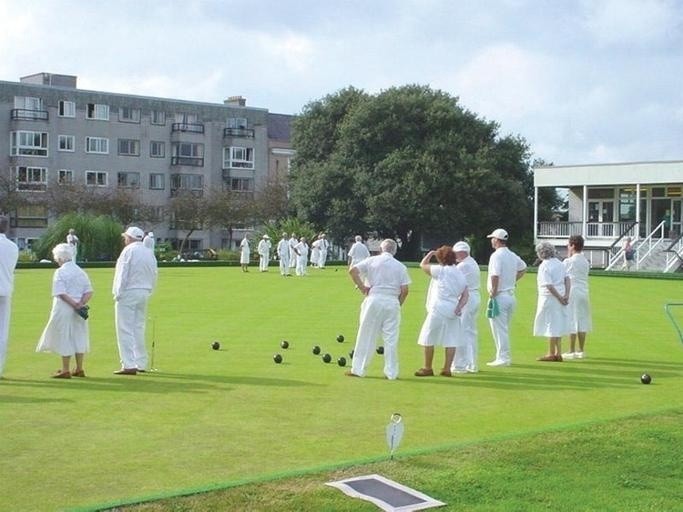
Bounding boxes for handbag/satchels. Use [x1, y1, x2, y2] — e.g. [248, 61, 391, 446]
[626, 253, 633, 260]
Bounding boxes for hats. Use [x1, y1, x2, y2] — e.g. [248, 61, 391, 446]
[121, 227, 142, 240]
[453, 241, 470, 252]
[487, 228, 509, 240]
[263, 235, 269, 238]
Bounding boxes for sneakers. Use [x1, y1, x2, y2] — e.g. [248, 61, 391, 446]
[51, 368, 84, 378]
[415, 368, 433, 376]
[536, 352, 585, 361]
[441, 366, 477, 376]
[113, 368, 144, 374]
[345, 370, 359, 376]
[487, 359, 510, 366]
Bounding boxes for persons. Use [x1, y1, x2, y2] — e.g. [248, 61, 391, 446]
[451, 241, 481, 373]
[415, 245, 469, 376]
[0, 215, 19, 369]
[238, 232, 250, 273]
[278, 232, 330, 276]
[112, 227, 157, 374]
[485, 229, 527, 367]
[619, 237, 634, 272]
[143, 232, 155, 249]
[661, 210, 671, 237]
[67, 229, 79, 263]
[348, 231, 383, 289]
[562, 234, 592, 359]
[532, 242, 571, 362]
[35, 243, 94, 378]
[344, 238, 411, 380]
[258, 235, 272, 272]
